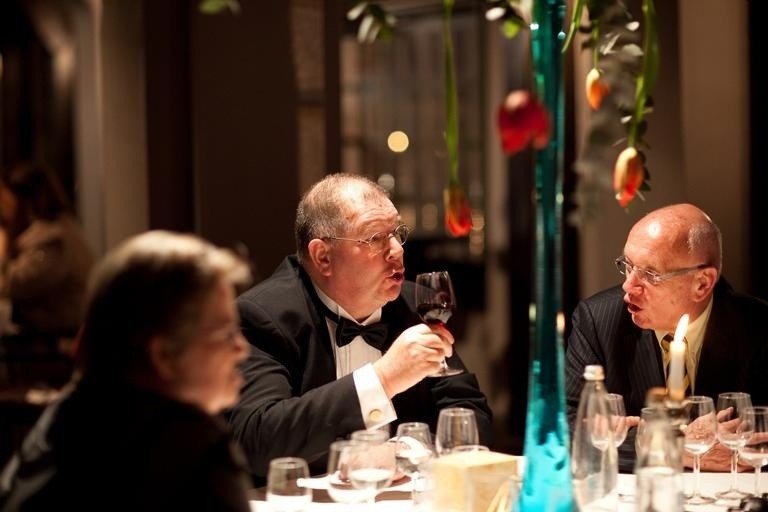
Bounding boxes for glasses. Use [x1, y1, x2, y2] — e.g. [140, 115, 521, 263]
[323, 224, 414, 254]
[616, 255, 708, 286]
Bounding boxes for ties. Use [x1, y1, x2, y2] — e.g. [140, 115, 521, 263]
[663, 334, 691, 402]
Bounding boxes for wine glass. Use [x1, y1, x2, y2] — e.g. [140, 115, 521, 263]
[413, 270, 464, 379]
[681, 391, 767, 507]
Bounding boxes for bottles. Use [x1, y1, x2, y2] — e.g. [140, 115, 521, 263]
[569, 365, 620, 512]
[635, 387, 683, 512]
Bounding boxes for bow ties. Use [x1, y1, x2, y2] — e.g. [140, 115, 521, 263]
[336, 316, 386, 350]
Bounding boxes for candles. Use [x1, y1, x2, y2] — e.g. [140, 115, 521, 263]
[669, 314, 689, 403]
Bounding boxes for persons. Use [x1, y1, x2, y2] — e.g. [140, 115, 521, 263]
[229, 172, 496, 492]
[0, 160, 97, 333]
[564, 202, 768, 476]
[1, 230, 254, 511]
[3, 248, 90, 480]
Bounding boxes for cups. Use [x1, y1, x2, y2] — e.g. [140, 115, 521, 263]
[590, 392, 627, 453]
[265, 408, 489, 512]
